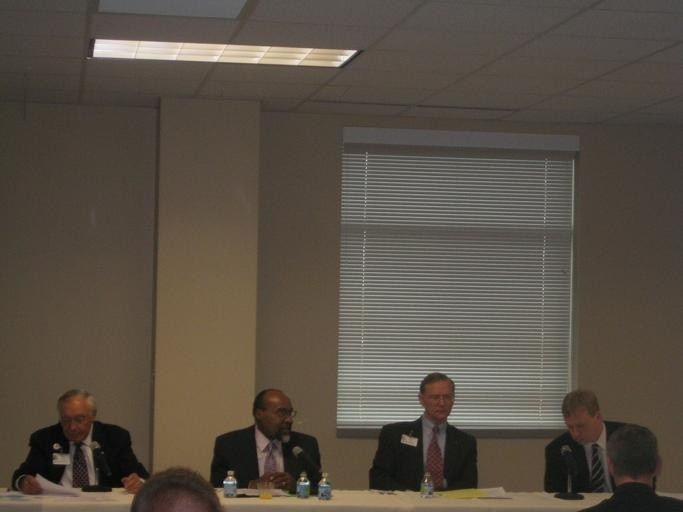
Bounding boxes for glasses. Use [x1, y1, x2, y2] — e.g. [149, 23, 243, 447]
[275, 407, 298, 422]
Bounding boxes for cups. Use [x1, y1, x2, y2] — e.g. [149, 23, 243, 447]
[256, 481, 275, 499]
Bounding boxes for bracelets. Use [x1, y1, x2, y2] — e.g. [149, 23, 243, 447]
[18, 477, 26, 491]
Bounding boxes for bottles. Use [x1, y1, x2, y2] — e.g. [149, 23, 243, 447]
[222, 469, 238, 499]
[296, 473, 310, 500]
[317, 472, 332, 501]
[419, 472, 434, 499]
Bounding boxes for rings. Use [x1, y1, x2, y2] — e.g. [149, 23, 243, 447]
[271, 477, 274, 480]
[283, 482, 286, 486]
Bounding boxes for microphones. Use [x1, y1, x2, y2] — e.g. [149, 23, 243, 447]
[90, 441, 112, 472]
[290, 445, 318, 474]
[560, 445, 583, 475]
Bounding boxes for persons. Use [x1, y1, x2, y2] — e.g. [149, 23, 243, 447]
[544, 390, 658, 493]
[369, 372, 478, 492]
[577, 425, 683, 511]
[12, 389, 150, 495]
[129, 466, 221, 512]
[210, 389, 323, 497]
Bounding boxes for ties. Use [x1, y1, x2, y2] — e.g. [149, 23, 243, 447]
[71, 439, 90, 490]
[424, 424, 446, 493]
[590, 441, 607, 494]
[264, 439, 277, 476]
